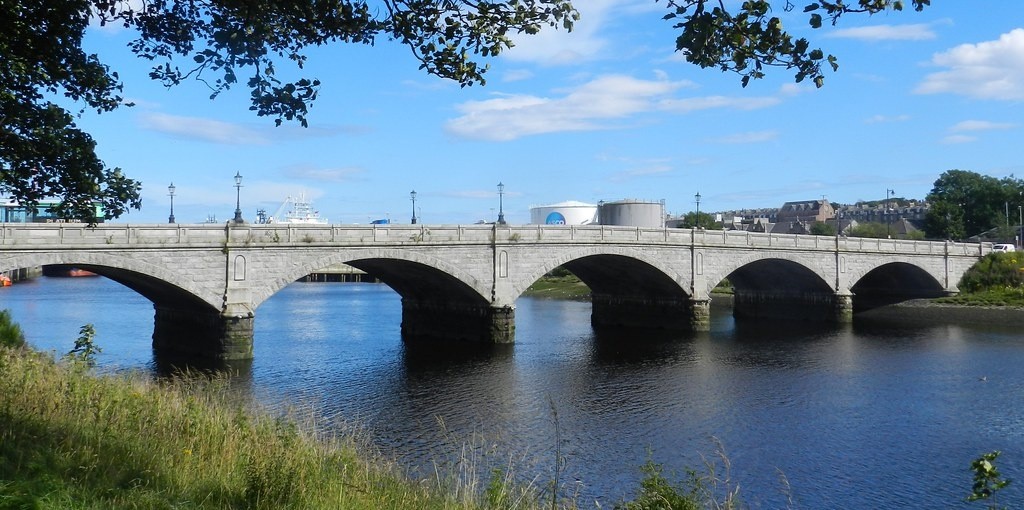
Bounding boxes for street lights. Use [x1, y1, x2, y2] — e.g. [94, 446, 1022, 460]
[887, 188, 895, 239]
[168, 181, 177, 224]
[694, 191, 702, 229]
[410, 189, 418, 224]
[233, 170, 244, 223]
[497, 181, 507, 225]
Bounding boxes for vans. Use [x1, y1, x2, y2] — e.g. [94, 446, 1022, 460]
[993, 244, 1015, 254]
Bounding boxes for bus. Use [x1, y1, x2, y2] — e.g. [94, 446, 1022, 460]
[0, 198, 105, 223]
